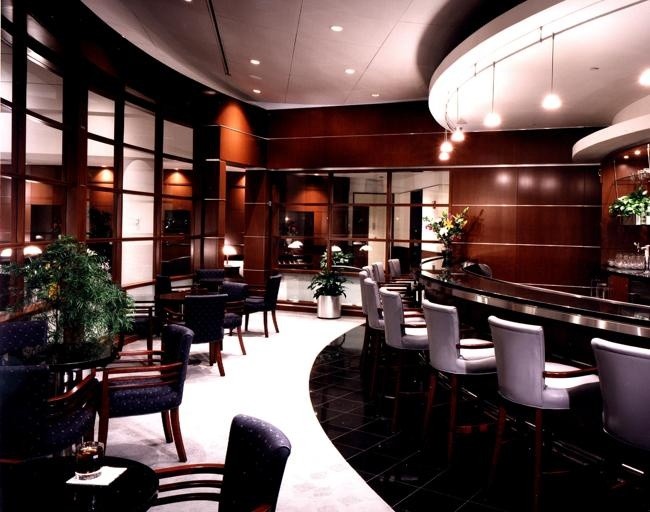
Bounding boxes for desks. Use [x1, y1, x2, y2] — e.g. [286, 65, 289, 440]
[1, 453, 160, 510]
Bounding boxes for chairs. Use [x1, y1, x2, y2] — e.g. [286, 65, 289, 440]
[591, 337, 649, 480]
[419, 298, 500, 460]
[486, 315, 603, 480]
[358, 256, 440, 432]
[0, 266, 292, 462]
[151, 414, 291, 510]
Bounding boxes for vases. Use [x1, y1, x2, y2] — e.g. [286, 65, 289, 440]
[619, 215, 636, 225]
[441, 251, 453, 265]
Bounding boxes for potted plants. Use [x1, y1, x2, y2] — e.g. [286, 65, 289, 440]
[306, 253, 353, 320]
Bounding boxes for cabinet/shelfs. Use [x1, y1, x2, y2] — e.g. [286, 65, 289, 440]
[601, 143, 649, 299]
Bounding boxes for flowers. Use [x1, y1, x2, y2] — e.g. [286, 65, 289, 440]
[608, 190, 650, 216]
[422, 205, 472, 251]
